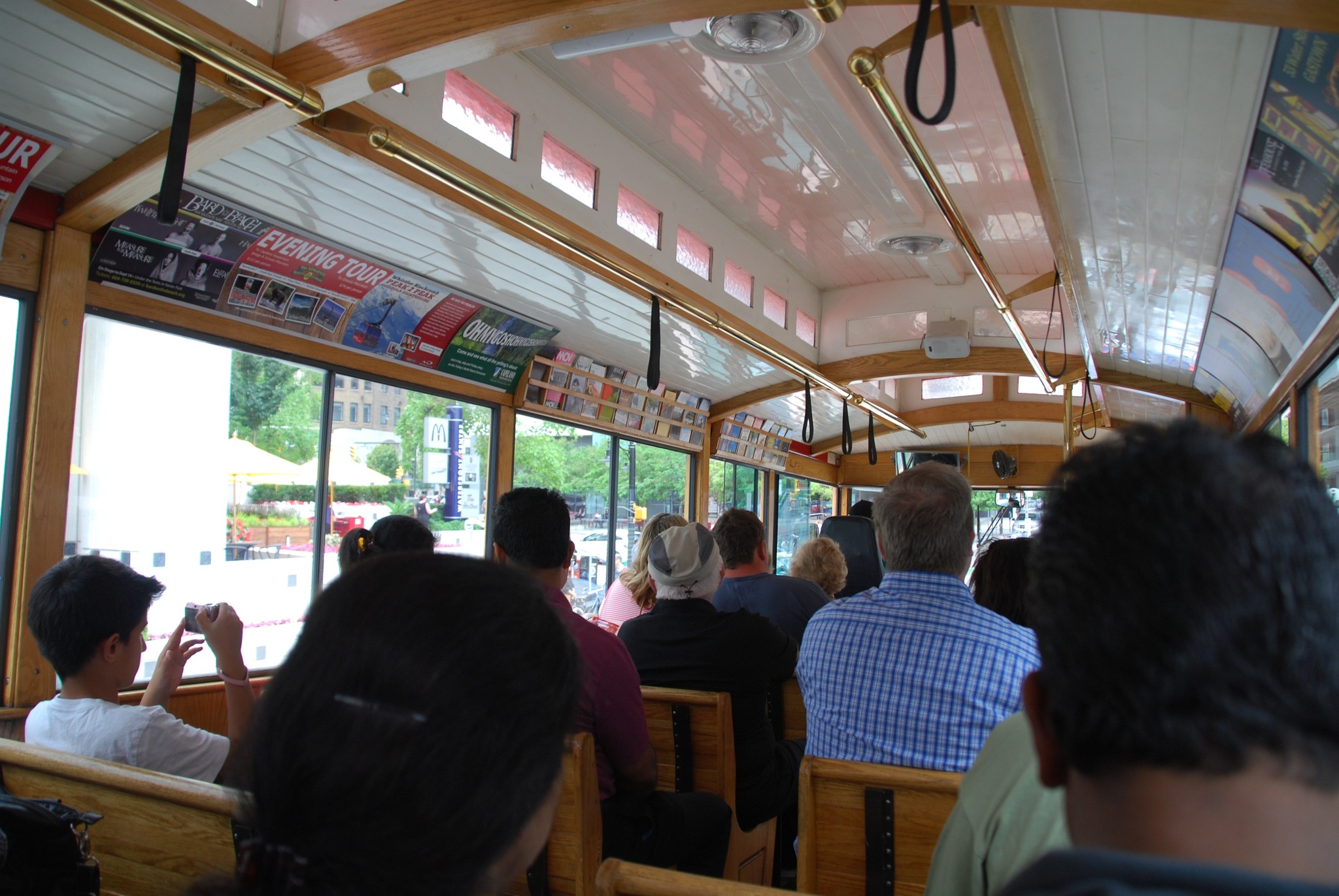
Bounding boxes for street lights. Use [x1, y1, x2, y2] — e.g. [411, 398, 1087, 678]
[600, 437, 637, 570]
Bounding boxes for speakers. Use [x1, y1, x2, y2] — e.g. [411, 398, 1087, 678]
[925, 317, 970, 359]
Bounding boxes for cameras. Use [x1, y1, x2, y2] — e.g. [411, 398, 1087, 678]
[184, 602, 219, 635]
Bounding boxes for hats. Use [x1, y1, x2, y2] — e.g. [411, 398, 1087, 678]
[647, 521, 720, 587]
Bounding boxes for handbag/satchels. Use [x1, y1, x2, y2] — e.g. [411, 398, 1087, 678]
[0, 767, 101, 895]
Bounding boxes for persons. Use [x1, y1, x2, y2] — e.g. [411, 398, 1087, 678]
[988, 417, 1339, 896]
[22, 555, 265, 788]
[412, 488, 448, 527]
[709, 507, 833, 680]
[794, 463, 1046, 781]
[596, 511, 691, 636]
[848, 500, 887, 572]
[1007, 498, 1019, 508]
[568, 500, 618, 527]
[788, 537, 849, 602]
[340, 516, 438, 573]
[190, 549, 590, 896]
[969, 536, 1054, 637]
[490, 487, 734, 883]
[146, 222, 239, 294]
[614, 520, 810, 891]
[925, 711, 1078, 896]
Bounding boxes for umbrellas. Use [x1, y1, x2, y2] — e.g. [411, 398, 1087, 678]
[195, 426, 307, 548]
[286, 445, 392, 535]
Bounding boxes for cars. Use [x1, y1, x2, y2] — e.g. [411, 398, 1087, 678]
[570, 531, 642, 566]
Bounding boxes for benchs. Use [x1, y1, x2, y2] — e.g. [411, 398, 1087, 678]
[0, 678, 970, 896]
